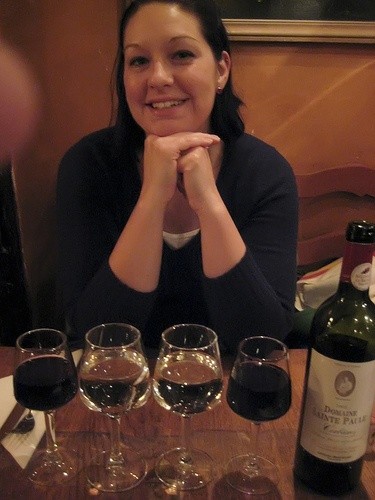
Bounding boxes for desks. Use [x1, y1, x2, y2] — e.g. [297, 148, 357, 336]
[1, 347, 375, 499]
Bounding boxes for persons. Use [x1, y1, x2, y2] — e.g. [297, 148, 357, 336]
[40, 0, 299, 357]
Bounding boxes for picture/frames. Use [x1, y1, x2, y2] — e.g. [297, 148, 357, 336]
[124, 0, 375, 44]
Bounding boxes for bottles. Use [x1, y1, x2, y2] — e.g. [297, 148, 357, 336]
[294, 222, 375, 495]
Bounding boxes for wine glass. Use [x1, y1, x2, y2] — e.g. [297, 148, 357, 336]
[13, 328, 83, 484]
[152, 324, 223, 490]
[224, 336, 292, 495]
[80, 324, 151, 491]
